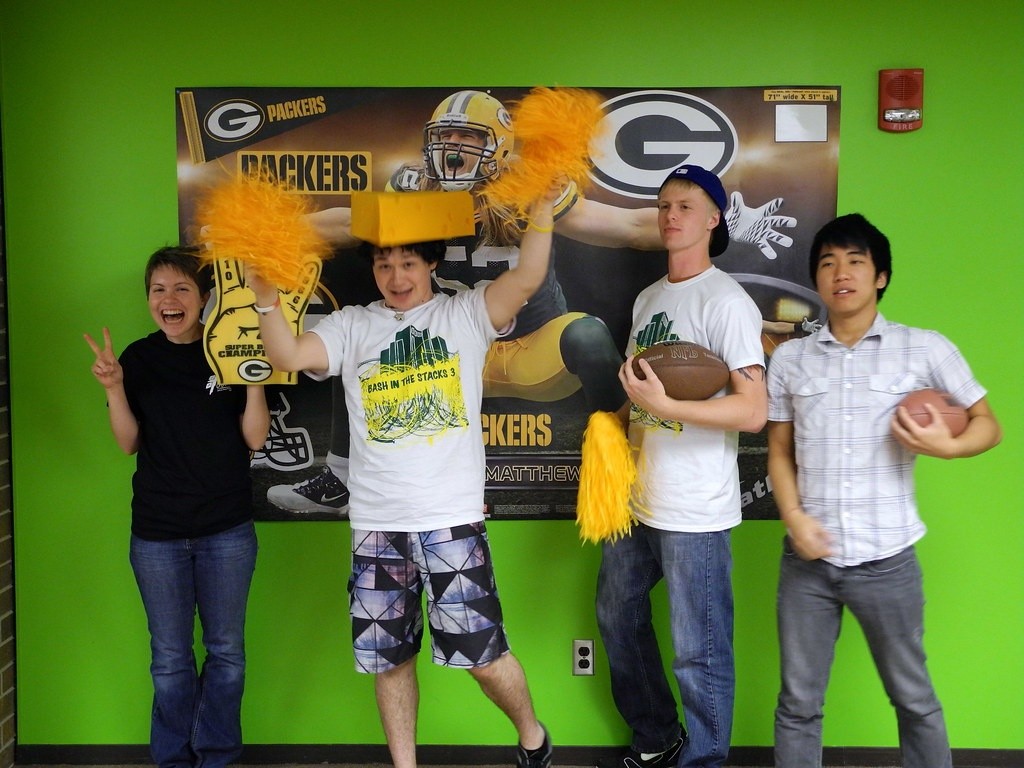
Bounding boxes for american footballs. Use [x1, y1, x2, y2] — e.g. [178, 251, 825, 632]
[892, 387, 973, 440]
[622, 340, 733, 403]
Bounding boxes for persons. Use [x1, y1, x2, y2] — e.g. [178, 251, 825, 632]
[766, 214, 1003, 768]
[201, 90, 823, 515]
[83, 244, 269, 768]
[594, 164, 766, 768]
[244, 171, 569, 768]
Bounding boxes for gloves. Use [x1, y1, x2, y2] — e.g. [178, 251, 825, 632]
[793, 316, 824, 334]
[723, 190, 797, 259]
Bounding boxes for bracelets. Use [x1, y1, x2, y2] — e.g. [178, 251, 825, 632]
[527, 219, 555, 233]
[253, 295, 280, 313]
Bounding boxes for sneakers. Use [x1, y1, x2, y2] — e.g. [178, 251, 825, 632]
[266, 464, 351, 515]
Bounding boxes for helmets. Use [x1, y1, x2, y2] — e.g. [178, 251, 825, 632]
[422, 89, 516, 193]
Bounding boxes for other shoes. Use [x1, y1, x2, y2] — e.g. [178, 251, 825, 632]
[515, 721, 553, 768]
[595, 723, 689, 768]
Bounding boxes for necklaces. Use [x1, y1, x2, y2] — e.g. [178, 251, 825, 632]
[390, 307, 405, 321]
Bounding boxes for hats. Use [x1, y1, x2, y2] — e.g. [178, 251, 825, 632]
[659, 163, 730, 258]
